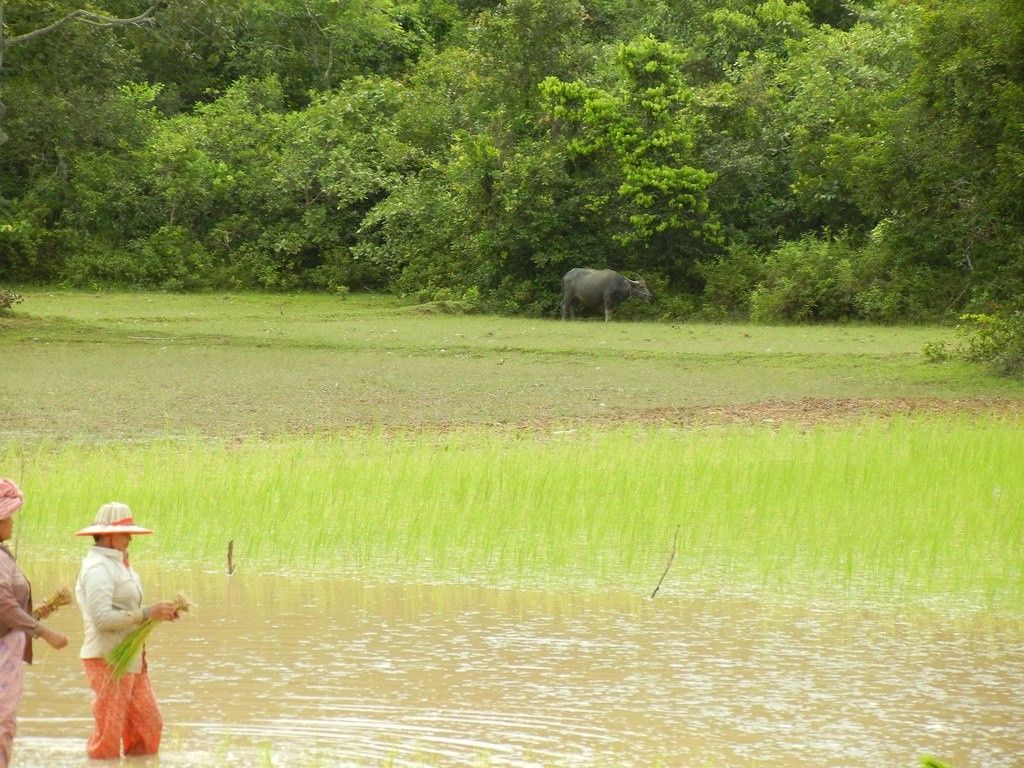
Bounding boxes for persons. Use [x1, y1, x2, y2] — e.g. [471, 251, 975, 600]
[78, 501, 182, 760]
[0, 478, 68, 768]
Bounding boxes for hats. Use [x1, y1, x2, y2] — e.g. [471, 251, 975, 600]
[74, 502, 154, 536]
[0, 478, 24, 520]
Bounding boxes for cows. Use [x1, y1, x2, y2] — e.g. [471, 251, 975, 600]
[560, 268, 652, 322]
